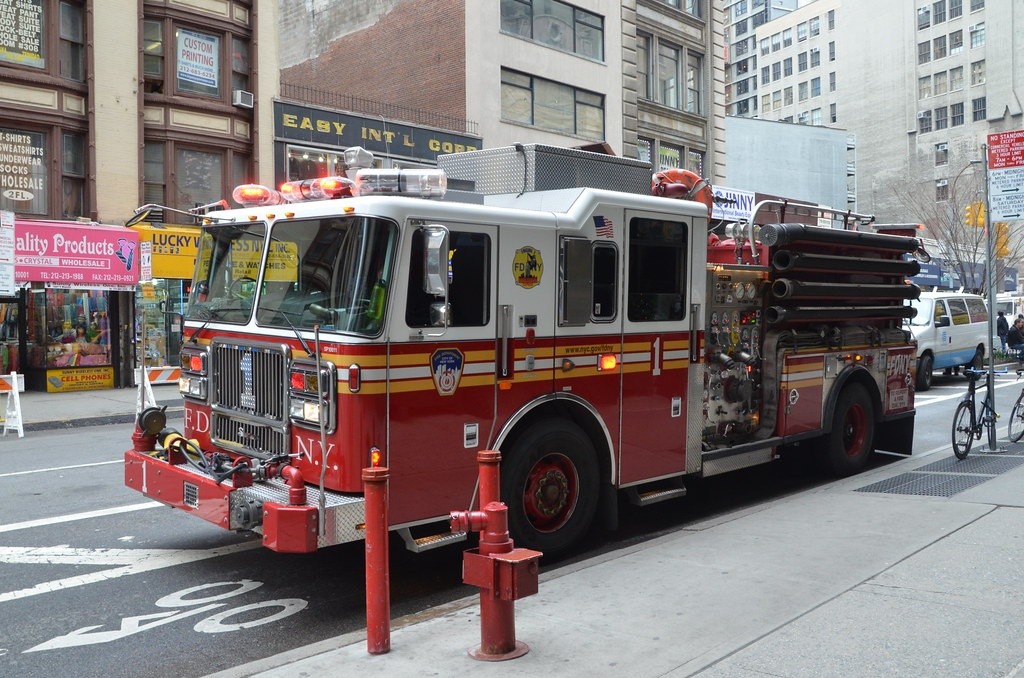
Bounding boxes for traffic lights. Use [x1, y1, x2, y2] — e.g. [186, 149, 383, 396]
[965, 203, 985, 227]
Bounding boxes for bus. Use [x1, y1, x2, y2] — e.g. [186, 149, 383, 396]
[985, 291, 1024, 329]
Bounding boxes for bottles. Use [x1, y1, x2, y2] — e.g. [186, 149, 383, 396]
[368, 278, 386, 321]
[194, 281, 209, 302]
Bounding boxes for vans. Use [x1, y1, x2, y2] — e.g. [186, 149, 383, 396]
[903, 291, 990, 391]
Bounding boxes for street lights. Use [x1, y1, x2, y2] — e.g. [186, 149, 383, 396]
[950, 161, 988, 291]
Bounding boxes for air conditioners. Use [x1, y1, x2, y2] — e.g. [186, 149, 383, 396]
[917, 111, 926, 119]
[921, 5, 930, 11]
[233, 89, 254, 109]
[969, 24, 977, 32]
[941, 179, 948, 185]
[939, 143, 948, 150]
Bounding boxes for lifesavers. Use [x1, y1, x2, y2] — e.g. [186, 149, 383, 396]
[650, 170, 714, 221]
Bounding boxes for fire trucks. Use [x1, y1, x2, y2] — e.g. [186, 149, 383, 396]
[122, 144, 931, 578]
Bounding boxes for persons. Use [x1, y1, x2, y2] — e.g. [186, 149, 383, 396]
[943, 366, 959, 375]
[86, 321, 103, 343]
[996, 311, 1010, 350]
[1008, 318, 1024, 360]
[75, 325, 87, 343]
[76, 314, 88, 331]
[61, 321, 75, 343]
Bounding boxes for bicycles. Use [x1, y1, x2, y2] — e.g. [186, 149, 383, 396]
[1006, 370, 1024, 443]
[951, 367, 1009, 460]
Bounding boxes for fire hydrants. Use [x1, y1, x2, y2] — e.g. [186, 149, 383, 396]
[450, 450, 543, 660]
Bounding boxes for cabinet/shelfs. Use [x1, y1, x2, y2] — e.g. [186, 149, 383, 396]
[136, 296, 166, 367]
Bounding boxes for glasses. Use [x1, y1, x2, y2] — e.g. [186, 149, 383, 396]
[1020, 322, 1024, 323]
